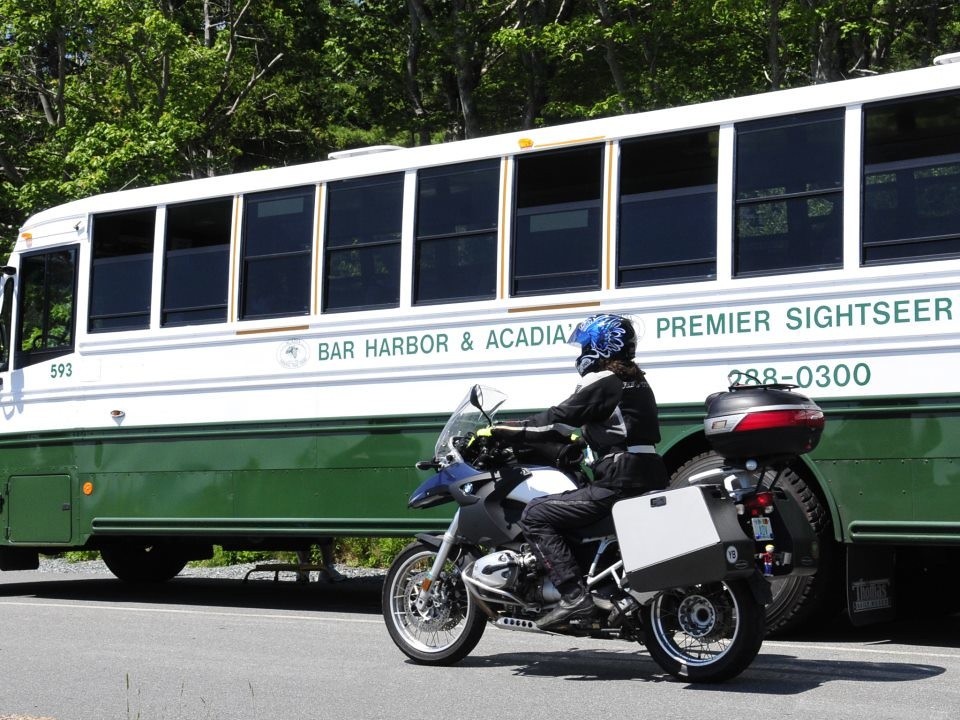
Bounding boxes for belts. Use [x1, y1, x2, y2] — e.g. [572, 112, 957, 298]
[627, 445, 656, 453]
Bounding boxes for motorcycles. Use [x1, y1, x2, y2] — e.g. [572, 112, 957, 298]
[382, 383, 825, 685]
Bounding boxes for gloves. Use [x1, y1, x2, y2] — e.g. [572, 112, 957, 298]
[570, 429, 588, 445]
[465, 425, 492, 450]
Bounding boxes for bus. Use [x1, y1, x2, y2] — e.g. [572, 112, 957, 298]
[1, 52, 960, 641]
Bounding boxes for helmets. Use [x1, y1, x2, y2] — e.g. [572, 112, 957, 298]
[575, 312, 636, 377]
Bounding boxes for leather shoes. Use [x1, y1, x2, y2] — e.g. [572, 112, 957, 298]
[536, 593, 595, 629]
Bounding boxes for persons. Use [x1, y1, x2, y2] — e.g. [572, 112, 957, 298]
[461, 313, 670, 627]
